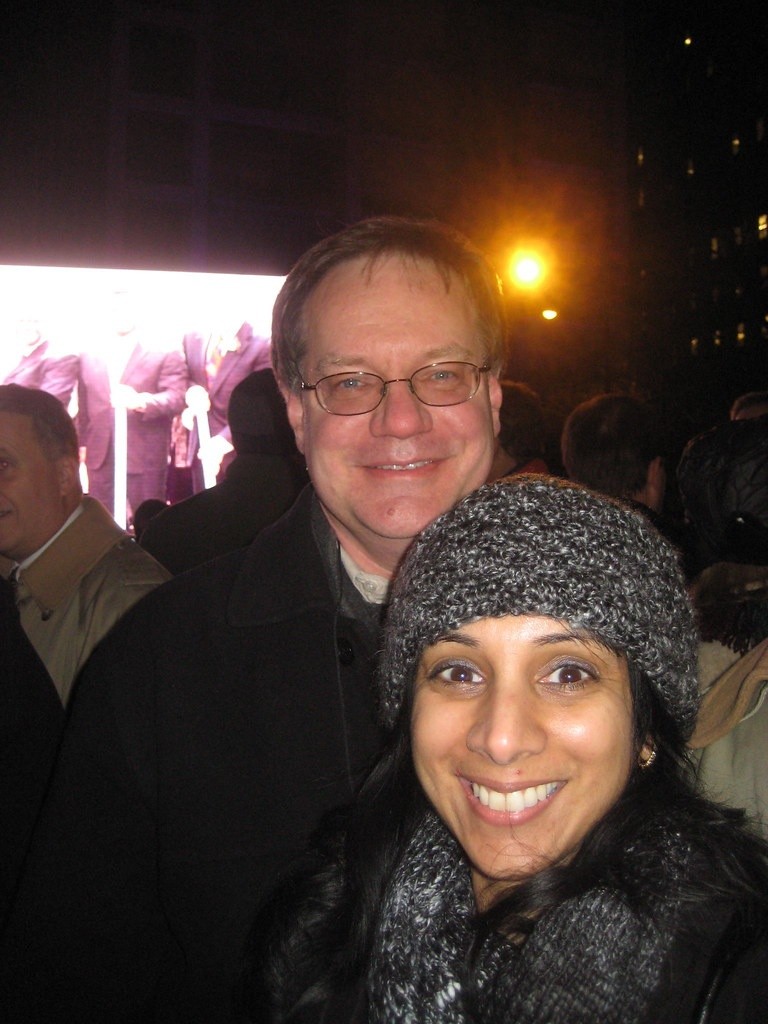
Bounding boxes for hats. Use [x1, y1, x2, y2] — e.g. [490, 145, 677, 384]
[374, 472, 700, 741]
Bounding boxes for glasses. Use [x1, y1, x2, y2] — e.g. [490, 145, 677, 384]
[301, 361, 491, 416]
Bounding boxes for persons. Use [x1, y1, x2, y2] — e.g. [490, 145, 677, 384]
[86, 317, 186, 537]
[2, 315, 86, 493]
[0, 215, 768, 1024]
[180, 307, 275, 497]
